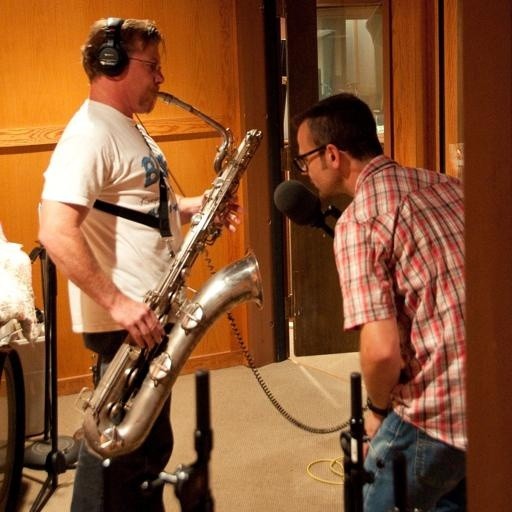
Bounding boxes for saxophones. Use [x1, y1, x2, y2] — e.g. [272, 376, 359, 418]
[75, 91, 264, 456]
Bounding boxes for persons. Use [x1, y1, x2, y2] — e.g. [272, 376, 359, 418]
[294, 94, 467, 511]
[37, 18, 241, 512]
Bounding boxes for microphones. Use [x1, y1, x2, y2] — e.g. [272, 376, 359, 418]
[272, 178, 327, 233]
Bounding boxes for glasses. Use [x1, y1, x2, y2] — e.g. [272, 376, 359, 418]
[128, 56, 161, 72]
[292, 142, 345, 172]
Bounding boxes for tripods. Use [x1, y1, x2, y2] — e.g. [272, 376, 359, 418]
[20, 251, 80, 512]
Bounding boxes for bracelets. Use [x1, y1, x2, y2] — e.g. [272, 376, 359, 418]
[366, 397, 386, 417]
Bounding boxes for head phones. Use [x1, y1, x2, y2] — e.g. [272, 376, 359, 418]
[97, 17, 129, 79]
